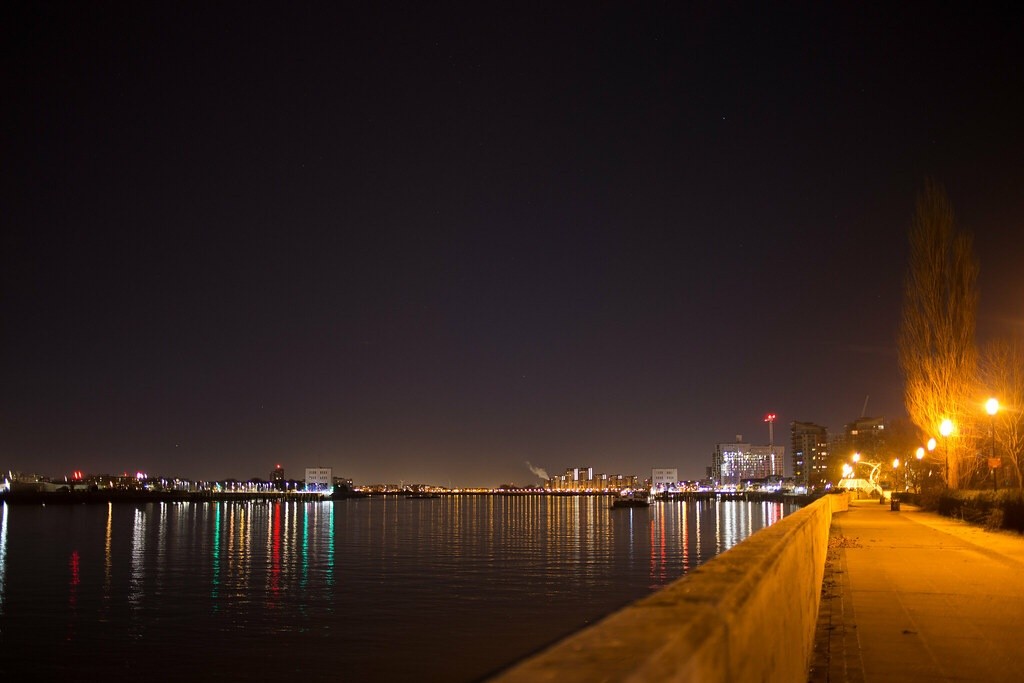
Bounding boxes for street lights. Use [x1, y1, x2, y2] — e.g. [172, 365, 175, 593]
[941, 420, 951, 488]
[985, 397, 1000, 492]
[916, 448, 924, 483]
[853, 453, 861, 500]
[893, 459, 900, 492]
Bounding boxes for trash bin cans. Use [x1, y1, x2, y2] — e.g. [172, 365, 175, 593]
[891, 497, 900, 511]
[880, 496, 885, 504]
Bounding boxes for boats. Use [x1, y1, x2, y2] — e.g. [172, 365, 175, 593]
[612, 487, 653, 508]
